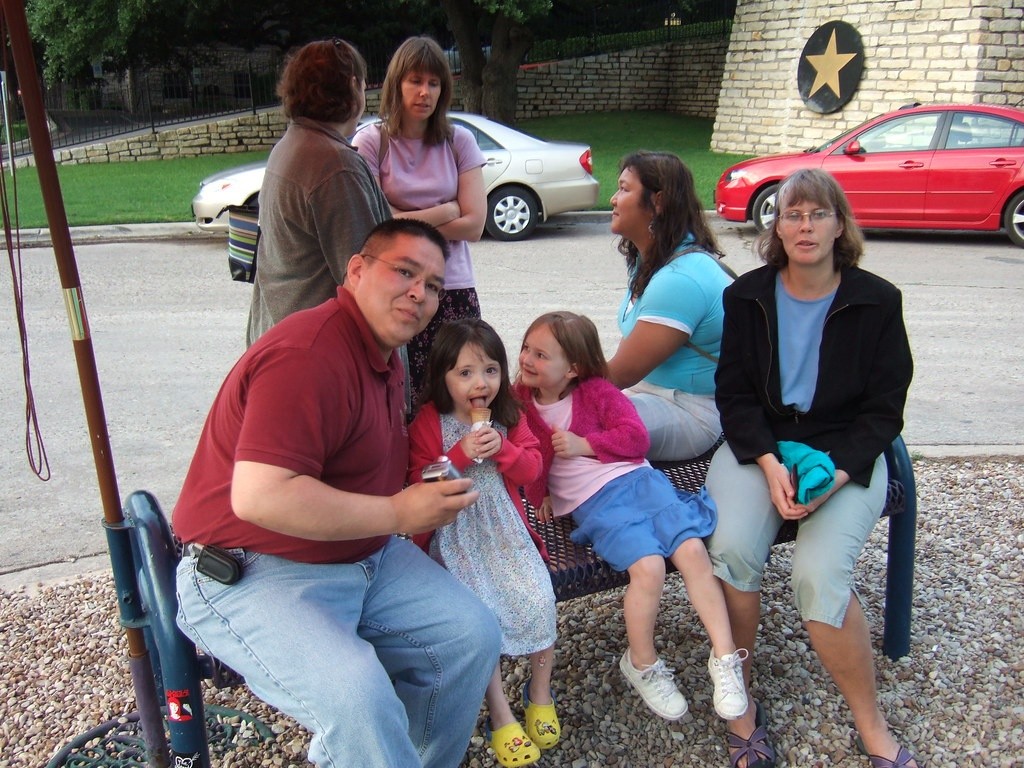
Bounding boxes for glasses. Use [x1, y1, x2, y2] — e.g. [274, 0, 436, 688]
[360, 253, 447, 301]
[777, 208, 838, 222]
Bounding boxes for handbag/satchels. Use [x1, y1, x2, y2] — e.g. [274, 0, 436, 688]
[215, 204, 261, 283]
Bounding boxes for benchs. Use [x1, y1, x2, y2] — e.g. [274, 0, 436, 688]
[125, 436, 916, 768]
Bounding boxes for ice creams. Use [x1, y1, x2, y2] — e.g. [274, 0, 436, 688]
[470, 407, 491, 424]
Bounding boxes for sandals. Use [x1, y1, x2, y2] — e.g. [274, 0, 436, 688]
[522, 678, 560, 750]
[485, 715, 541, 768]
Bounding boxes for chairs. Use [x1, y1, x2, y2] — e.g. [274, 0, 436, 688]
[957, 122, 973, 147]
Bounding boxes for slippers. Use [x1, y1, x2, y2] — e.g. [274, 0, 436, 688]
[725, 703, 775, 768]
[856, 733, 918, 768]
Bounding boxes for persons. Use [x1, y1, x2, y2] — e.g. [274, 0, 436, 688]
[604, 151, 738, 463]
[703, 167, 918, 768]
[410, 318, 561, 768]
[171, 217, 502, 768]
[247, 38, 394, 355]
[350, 35, 490, 427]
[508, 310, 749, 721]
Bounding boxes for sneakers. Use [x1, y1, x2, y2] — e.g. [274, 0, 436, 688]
[619, 646, 688, 721]
[707, 646, 750, 721]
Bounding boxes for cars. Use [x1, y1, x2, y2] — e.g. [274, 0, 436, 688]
[713, 100, 1024, 249]
[190, 110, 600, 241]
[441, 38, 498, 74]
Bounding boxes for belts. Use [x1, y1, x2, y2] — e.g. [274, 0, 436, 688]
[183, 544, 201, 557]
